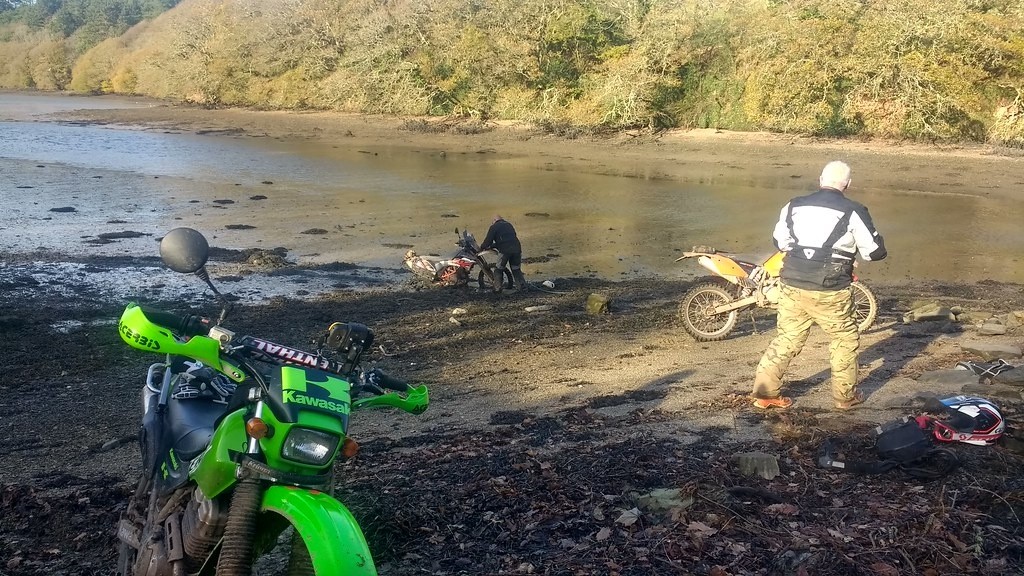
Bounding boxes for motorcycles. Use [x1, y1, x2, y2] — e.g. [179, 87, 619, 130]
[401, 226, 514, 293]
[673, 245, 878, 343]
[117, 227, 428, 576]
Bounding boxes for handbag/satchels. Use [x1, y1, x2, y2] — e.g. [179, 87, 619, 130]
[876, 423, 928, 462]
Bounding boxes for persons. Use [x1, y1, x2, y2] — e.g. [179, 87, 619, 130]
[751, 160, 887, 410]
[479, 214, 527, 291]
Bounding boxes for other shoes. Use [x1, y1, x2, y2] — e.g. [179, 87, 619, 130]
[834, 388, 866, 408]
[753, 395, 792, 409]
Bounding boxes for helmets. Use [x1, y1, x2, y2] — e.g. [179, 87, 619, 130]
[933, 395, 1005, 446]
